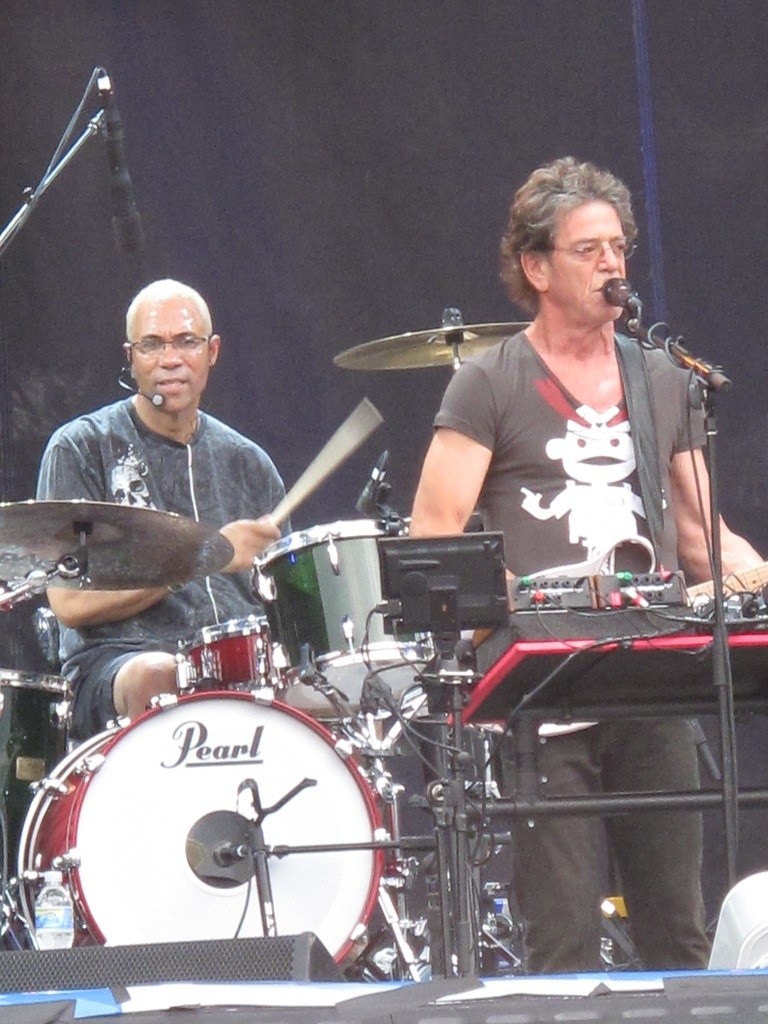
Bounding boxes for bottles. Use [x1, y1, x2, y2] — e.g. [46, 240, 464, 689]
[485, 902, 511, 979]
[36, 870, 75, 950]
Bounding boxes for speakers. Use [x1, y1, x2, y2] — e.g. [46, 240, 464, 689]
[0, 931, 350, 991]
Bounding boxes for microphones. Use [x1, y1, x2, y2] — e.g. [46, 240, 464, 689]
[94, 77, 144, 252]
[213, 841, 245, 867]
[118, 367, 164, 406]
[602, 277, 645, 312]
[356, 451, 390, 518]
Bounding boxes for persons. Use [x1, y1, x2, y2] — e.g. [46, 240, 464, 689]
[408, 160, 767, 972]
[36, 278, 294, 736]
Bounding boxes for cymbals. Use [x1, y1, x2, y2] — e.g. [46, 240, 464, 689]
[333, 321, 532, 368]
[0, 498, 234, 590]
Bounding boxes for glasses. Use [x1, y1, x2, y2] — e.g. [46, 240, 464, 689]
[553, 234, 635, 261]
[127, 334, 210, 356]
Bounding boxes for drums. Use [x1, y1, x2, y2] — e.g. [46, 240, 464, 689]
[16, 691, 385, 969]
[0, 670, 70, 888]
[176, 615, 289, 699]
[251, 519, 435, 718]
